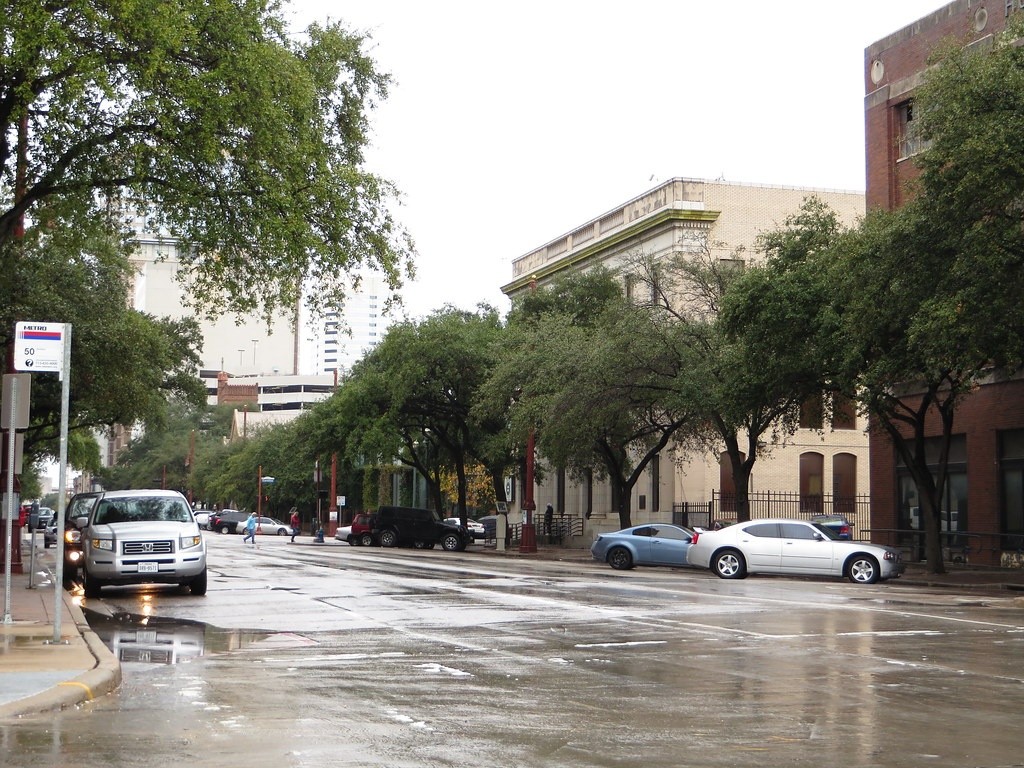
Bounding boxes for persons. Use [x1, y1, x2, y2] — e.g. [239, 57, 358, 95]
[290, 511, 302, 543]
[544, 503, 555, 536]
[242, 512, 258, 545]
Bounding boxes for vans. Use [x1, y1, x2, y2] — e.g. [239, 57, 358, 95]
[77, 490, 207, 598]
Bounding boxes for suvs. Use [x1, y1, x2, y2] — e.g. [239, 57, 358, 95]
[53, 492, 104, 583]
[352, 513, 378, 547]
[371, 506, 475, 551]
[210, 511, 249, 534]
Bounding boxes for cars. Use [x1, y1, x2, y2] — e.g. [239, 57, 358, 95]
[812, 514, 855, 540]
[198, 512, 216, 530]
[334, 526, 352, 543]
[235, 516, 293, 536]
[445, 518, 484, 536]
[44, 516, 58, 548]
[591, 523, 708, 570]
[88, 620, 203, 671]
[28, 509, 52, 532]
[687, 519, 902, 584]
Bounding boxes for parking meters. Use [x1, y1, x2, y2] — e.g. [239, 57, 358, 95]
[25, 514, 39, 589]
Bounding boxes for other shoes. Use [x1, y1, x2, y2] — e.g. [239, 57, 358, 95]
[244, 539, 246, 543]
[251, 542, 256, 544]
[291, 537, 294, 542]
